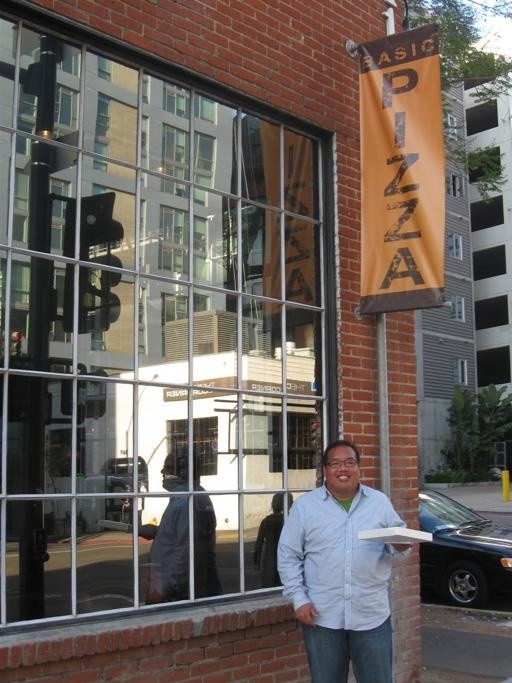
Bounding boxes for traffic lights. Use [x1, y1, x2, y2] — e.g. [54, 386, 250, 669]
[10, 329, 21, 361]
[63, 192, 122, 334]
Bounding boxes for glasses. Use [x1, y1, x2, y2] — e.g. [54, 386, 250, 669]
[325, 459, 359, 467]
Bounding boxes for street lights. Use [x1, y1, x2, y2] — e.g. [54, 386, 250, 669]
[125, 374, 160, 469]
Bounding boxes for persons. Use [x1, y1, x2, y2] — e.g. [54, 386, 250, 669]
[277, 442, 410, 683]
[254, 492, 295, 587]
[138, 449, 221, 604]
[45, 478, 55, 535]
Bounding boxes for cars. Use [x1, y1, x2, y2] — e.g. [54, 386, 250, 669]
[418, 489, 512, 607]
